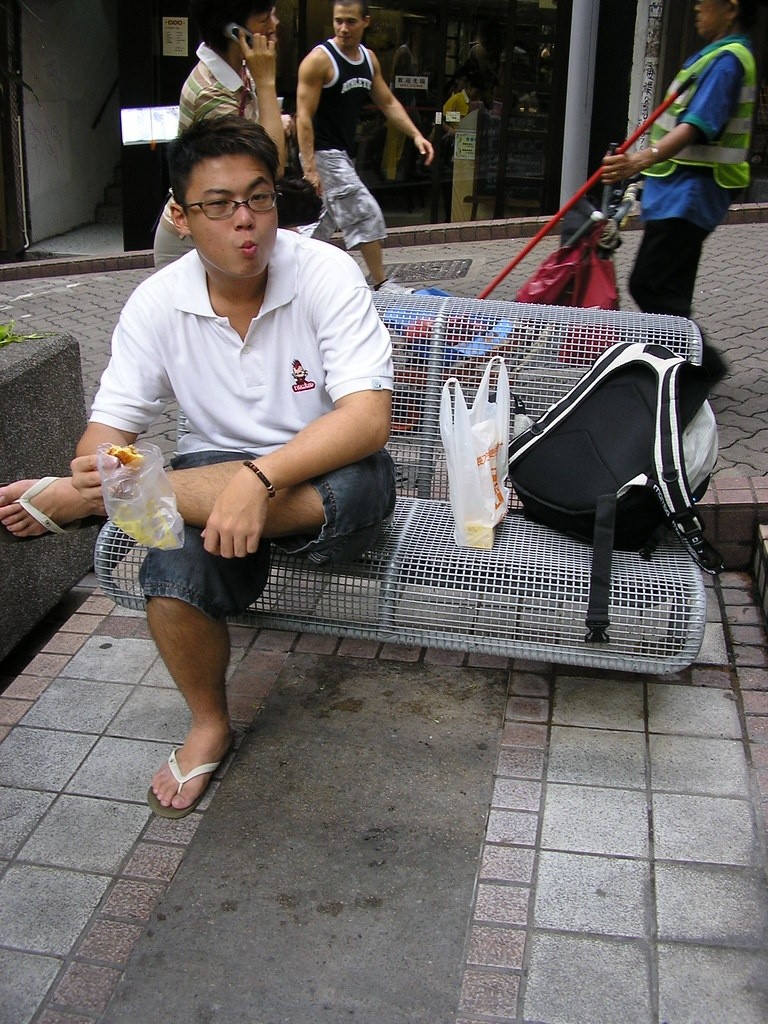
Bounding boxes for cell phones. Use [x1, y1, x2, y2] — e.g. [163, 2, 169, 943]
[224, 23, 253, 47]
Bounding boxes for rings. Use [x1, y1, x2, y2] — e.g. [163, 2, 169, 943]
[612, 172, 618, 176]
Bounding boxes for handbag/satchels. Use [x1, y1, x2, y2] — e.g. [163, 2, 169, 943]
[439, 357, 512, 549]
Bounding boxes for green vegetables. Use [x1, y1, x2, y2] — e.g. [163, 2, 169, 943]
[0, 320, 46, 347]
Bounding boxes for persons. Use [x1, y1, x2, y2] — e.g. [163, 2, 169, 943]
[295, 0, 437, 295]
[153, 0, 295, 274]
[599, 0, 761, 357]
[388, 27, 423, 131]
[0, 114, 399, 819]
[440, 74, 487, 223]
[454, 31, 503, 102]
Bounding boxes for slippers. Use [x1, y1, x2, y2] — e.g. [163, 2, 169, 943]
[0, 476, 108, 543]
[147, 746, 221, 819]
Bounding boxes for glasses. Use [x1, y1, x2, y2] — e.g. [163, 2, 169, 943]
[187, 190, 276, 219]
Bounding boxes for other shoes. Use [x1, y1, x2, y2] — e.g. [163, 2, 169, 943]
[374, 278, 416, 295]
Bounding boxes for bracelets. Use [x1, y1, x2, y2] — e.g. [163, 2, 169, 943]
[242, 459, 275, 498]
[650, 144, 660, 164]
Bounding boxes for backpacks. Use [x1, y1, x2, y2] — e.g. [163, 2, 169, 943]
[507, 340, 728, 645]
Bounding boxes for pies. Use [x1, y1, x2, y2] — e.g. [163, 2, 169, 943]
[106, 447, 143, 467]
